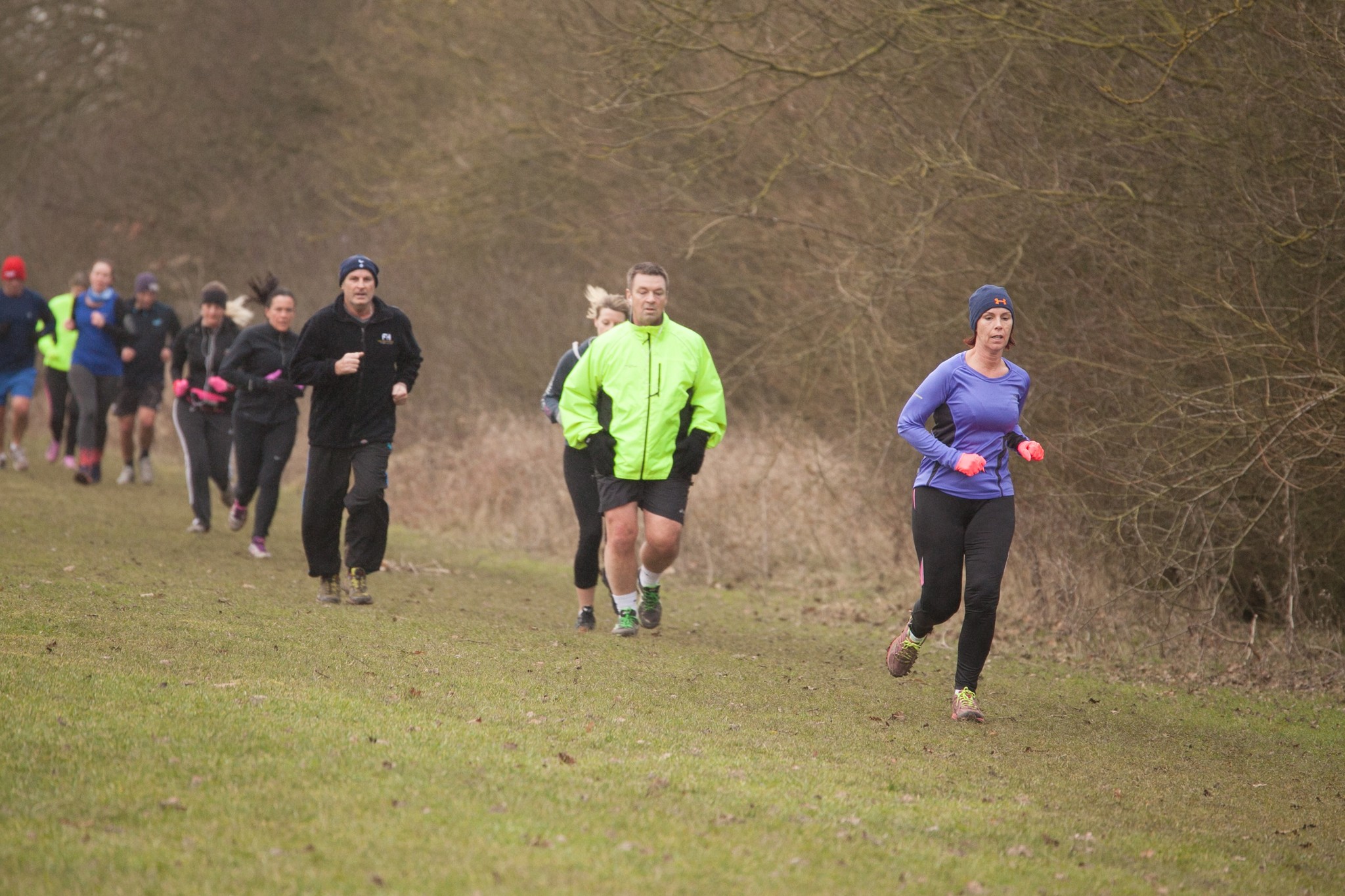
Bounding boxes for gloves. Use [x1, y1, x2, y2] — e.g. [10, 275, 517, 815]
[955, 452, 986, 477]
[583, 428, 618, 476]
[1017, 440, 1045, 462]
[248, 376, 304, 399]
[677, 428, 711, 475]
[173, 378, 189, 398]
[208, 375, 228, 394]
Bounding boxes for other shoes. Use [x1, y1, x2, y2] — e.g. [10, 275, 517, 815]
[116, 457, 154, 486]
[0, 442, 30, 473]
[46, 438, 75, 469]
[72, 462, 101, 485]
[213, 479, 236, 508]
[186, 517, 210, 533]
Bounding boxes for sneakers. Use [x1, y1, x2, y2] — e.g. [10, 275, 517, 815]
[345, 567, 374, 604]
[316, 573, 342, 607]
[575, 605, 596, 633]
[886, 610, 928, 678]
[636, 566, 663, 629]
[248, 535, 271, 558]
[228, 498, 249, 532]
[612, 607, 641, 638]
[952, 687, 985, 724]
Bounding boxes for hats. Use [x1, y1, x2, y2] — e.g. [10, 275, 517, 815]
[968, 284, 1015, 334]
[200, 280, 228, 310]
[338, 254, 379, 288]
[2, 255, 27, 281]
[134, 271, 159, 295]
